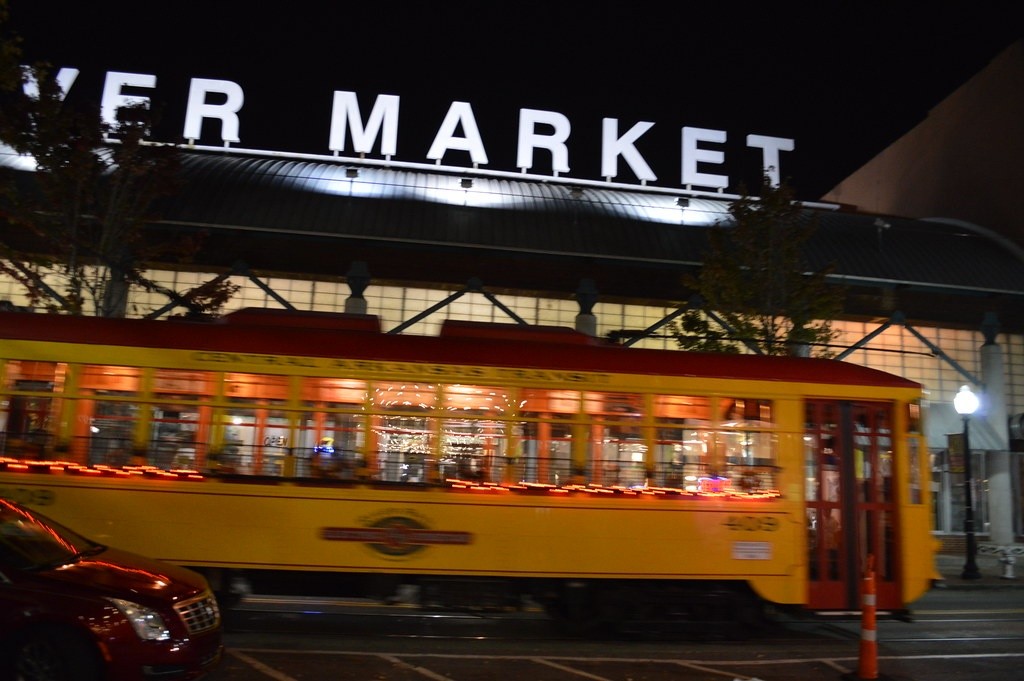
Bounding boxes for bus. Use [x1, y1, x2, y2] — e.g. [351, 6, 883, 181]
[0, 297, 946, 644]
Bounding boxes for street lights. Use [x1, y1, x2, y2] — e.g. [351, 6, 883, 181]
[953, 385, 982, 580]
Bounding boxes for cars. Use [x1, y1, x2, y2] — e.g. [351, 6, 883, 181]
[0, 492, 226, 681]
